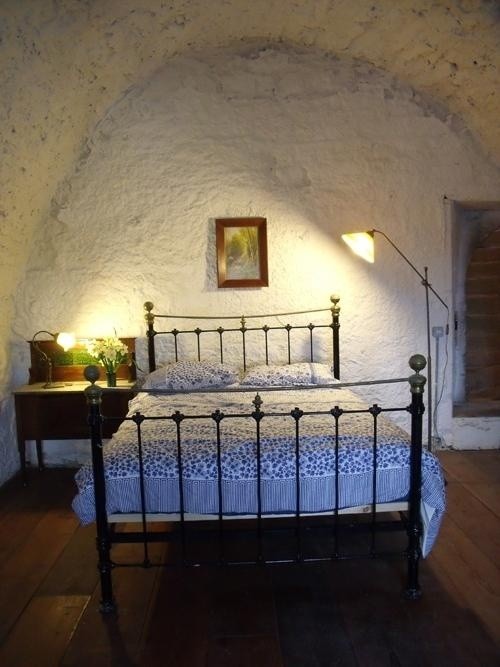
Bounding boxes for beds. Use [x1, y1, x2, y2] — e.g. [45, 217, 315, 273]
[83, 294, 427, 616]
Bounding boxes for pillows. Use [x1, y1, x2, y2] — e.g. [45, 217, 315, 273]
[141, 359, 241, 391]
[240, 362, 341, 388]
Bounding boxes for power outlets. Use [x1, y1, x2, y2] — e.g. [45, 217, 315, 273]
[432, 327, 444, 337]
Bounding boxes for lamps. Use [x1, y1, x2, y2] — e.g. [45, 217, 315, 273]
[341, 228, 450, 487]
[32, 330, 76, 389]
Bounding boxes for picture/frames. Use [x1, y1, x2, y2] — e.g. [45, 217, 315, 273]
[215, 217, 269, 288]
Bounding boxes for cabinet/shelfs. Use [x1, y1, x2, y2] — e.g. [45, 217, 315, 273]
[11, 338, 137, 472]
